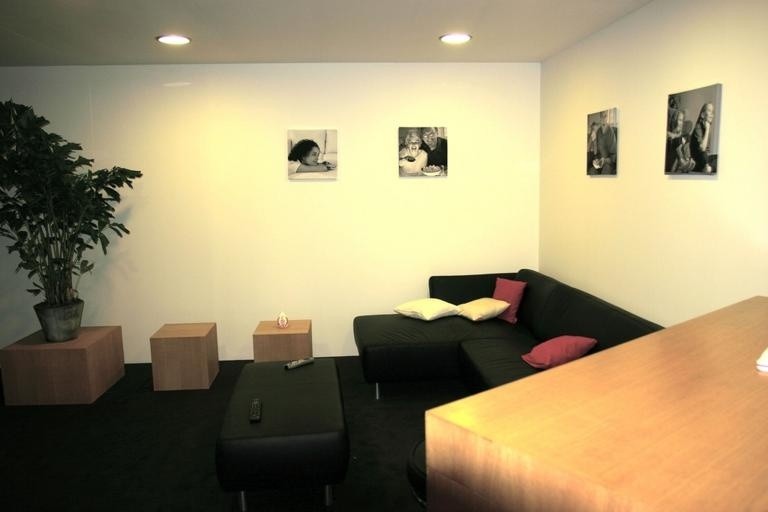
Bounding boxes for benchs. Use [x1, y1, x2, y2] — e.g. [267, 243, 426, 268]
[213, 358, 350, 510]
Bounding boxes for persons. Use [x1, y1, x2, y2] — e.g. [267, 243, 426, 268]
[399, 128, 447, 173]
[288, 138, 332, 174]
[666, 108, 695, 173]
[589, 110, 617, 175]
[690, 102, 717, 173]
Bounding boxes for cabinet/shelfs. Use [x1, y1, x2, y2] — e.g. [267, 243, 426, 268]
[1, 323, 128, 410]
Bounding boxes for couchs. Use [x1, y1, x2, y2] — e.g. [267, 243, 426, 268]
[353, 266, 671, 401]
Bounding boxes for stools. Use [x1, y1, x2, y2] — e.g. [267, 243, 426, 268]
[148, 320, 221, 398]
[250, 318, 314, 363]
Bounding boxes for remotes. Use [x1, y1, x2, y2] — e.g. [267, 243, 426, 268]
[285, 356, 314, 370]
[249, 398, 261, 420]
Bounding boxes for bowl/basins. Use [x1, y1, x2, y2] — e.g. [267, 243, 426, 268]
[420, 167, 441, 176]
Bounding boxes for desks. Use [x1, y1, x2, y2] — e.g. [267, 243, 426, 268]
[422, 293, 768, 511]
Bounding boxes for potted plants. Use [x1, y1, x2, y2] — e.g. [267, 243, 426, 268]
[0, 97, 145, 345]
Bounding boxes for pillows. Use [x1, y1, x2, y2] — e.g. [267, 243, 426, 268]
[522, 333, 597, 372]
[394, 296, 460, 323]
[493, 277, 528, 326]
[457, 293, 511, 325]
